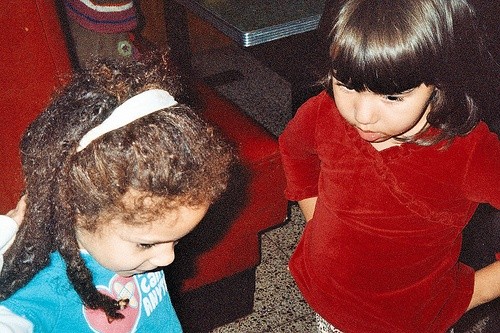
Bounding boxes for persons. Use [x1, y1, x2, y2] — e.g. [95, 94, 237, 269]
[0, 50, 240, 333]
[277, 0, 500, 333]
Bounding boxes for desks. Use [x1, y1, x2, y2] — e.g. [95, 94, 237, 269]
[178, 0, 328, 119]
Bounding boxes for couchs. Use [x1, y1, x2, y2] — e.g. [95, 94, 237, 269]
[1, 0, 288, 332]
